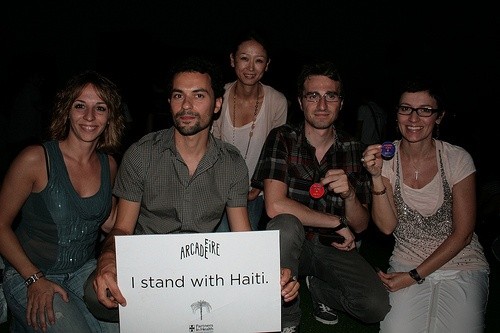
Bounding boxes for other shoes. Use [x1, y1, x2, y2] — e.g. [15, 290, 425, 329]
[281, 326, 298, 332]
[305, 275, 338, 325]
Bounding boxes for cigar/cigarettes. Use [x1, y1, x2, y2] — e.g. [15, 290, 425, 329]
[288, 276, 296, 283]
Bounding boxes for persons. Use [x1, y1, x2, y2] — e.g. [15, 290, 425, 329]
[0, 69, 125, 333]
[361, 81, 490, 333]
[250, 66, 392, 333]
[210, 38, 288, 233]
[83, 64, 300, 324]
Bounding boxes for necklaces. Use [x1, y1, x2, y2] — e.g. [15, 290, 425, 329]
[233, 84, 259, 161]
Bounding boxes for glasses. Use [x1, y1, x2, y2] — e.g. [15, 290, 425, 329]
[397, 105, 441, 117]
[303, 92, 343, 103]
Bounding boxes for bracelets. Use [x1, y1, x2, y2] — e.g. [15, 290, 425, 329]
[24, 270, 44, 287]
[372, 186, 387, 195]
[409, 268, 425, 285]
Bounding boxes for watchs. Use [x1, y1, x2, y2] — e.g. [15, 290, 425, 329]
[332, 217, 348, 232]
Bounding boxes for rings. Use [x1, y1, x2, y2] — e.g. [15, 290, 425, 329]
[361, 157, 365, 162]
